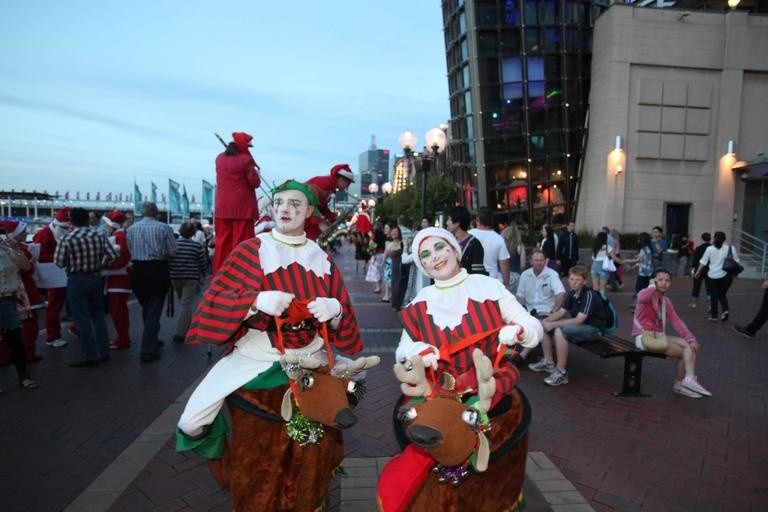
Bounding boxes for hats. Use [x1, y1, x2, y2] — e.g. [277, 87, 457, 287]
[53, 207, 72, 226]
[102, 211, 126, 230]
[411, 227, 463, 278]
[0, 221, 27, 238]
[271, 179, 322, 225]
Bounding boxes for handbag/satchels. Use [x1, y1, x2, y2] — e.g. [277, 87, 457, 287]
[642, 331, 668, 354]
[593, 290, 618, 333]
[723, 257, 744, 274]
[602, 256, 617, 273]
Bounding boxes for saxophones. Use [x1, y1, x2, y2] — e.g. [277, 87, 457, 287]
[316, 191, 363, 247]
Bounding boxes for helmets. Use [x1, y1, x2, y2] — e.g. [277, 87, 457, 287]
[336, 164, 355, 184]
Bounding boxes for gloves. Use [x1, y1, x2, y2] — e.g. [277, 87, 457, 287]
[256, 290, 296, 317]
[306, 297, 341, 323]
[498, 323, 529, 346]
[409, 341, 440, 371]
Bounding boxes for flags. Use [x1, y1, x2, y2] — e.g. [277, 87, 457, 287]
[135, 179, 215, 218]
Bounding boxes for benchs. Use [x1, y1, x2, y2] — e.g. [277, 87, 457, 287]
[574, 332, 666, 398]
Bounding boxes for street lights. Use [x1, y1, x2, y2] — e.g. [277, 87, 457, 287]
[346, 199, 377, 233]
[368, 181, 391, 220]
[399, 127, 445, 218]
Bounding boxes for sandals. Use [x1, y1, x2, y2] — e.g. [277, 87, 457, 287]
[22, 378, 39, 389]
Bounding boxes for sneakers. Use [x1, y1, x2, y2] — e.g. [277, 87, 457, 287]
[46, 338, 68, 348]
[682, 377, 712, 398]
[504, 353, 524, 368]
[108, 339, 132, 350]
[732, 324, 754, 340]
[672, 380, 704, 399]
[688, 303, 696, 309]
[173, 334, 184, 343]
[528, 358, 554, 373]
[29, 355, 43, 365]
[544, 367, 569, 387]
[707, 306, 729, 321]
[140, 340, 164, 362]
[69, 357, 100, 369]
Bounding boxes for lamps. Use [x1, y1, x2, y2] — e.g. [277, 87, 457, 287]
[728, 140, 735, 153]
[616, 135, 623, 150]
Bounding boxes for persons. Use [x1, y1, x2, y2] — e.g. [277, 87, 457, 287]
[513, 213, 768, 399]
[1, 202, 215, 390]
[185, 131, 545, 511]
